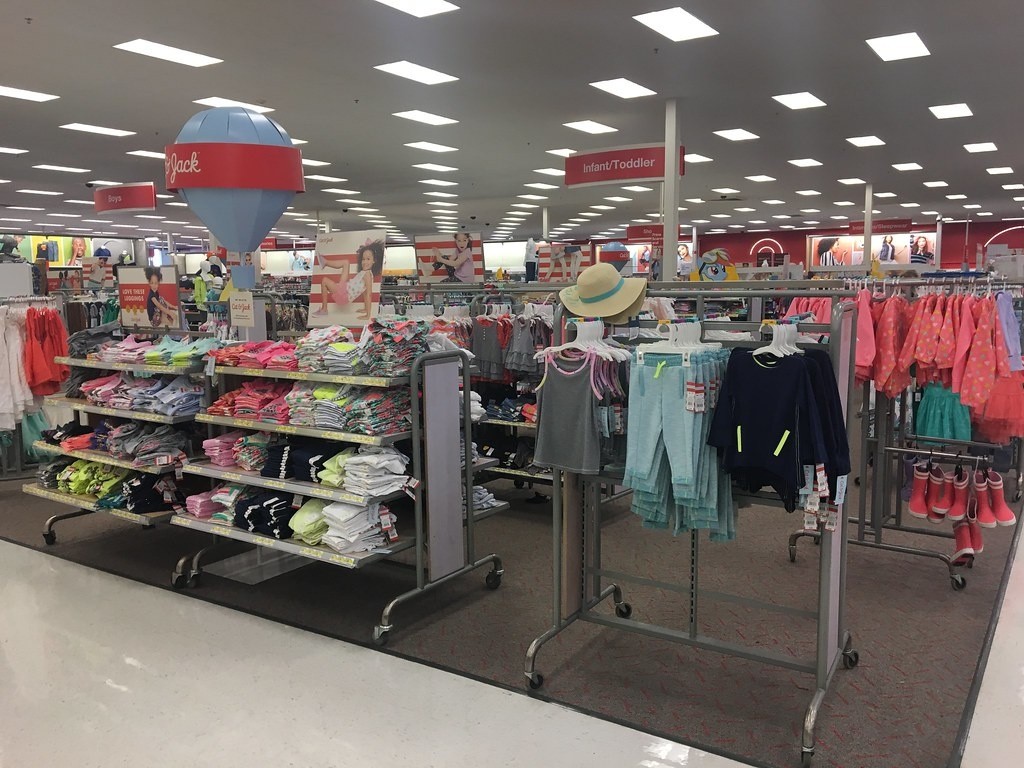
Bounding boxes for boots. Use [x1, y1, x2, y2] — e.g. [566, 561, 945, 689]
[902, 453, 1017, 565]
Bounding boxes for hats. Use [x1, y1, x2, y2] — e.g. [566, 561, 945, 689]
[559, 262, 648, 325]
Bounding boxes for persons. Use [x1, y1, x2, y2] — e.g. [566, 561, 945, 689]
[544, 245, 568, 283]
[179, 275, 194, 290]
[65, 237, 87, 267]
[639, 246, 650, 271]
[911, 236, 934, 264]
[879, 235, 895, 263]
[418, 232, 474, 283]
[118, 250, 132, 266]
[143, 266, 179, 327]
[195, 261, 216, 322]
[93, 245, 112, 257]
[0, 234, 25, 253]
[311, 239, 385, 321]
[564, 246, 583, 282]
[677, 244, 692, 263]
[522, 238, 536, 283]
[291, 251, 305, 272]
[88, 257, 108, 288]
[244, 253, 252, 266]
[817, 238, 848, 266]
[196, 251, 222, 278]
[207, 277, 226, 312]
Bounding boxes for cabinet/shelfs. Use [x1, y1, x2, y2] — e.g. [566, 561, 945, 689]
[23, 351, 563, 570]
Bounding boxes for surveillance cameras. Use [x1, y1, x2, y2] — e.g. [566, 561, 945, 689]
[461, 226, 466, 229]
[86, 183, 94, 187]
[486, 223, 490, 226]
[721, 196, 726, 199]
[470, 216, 476, 219]
[343, 209, 348, 212]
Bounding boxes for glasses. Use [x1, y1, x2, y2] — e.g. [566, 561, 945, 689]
[246, 258, 251, 261]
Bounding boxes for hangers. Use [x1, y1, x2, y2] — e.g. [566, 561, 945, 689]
[205, 311, 228, 325]
[0, 289, 120, 323]
[809, 274, 1024, 315]
[475, 302, 823, 367]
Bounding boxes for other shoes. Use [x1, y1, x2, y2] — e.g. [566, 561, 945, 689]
[312, 307, 328, 317]
[317, 252, 326, 270]
[419, 258, 434, 277]
